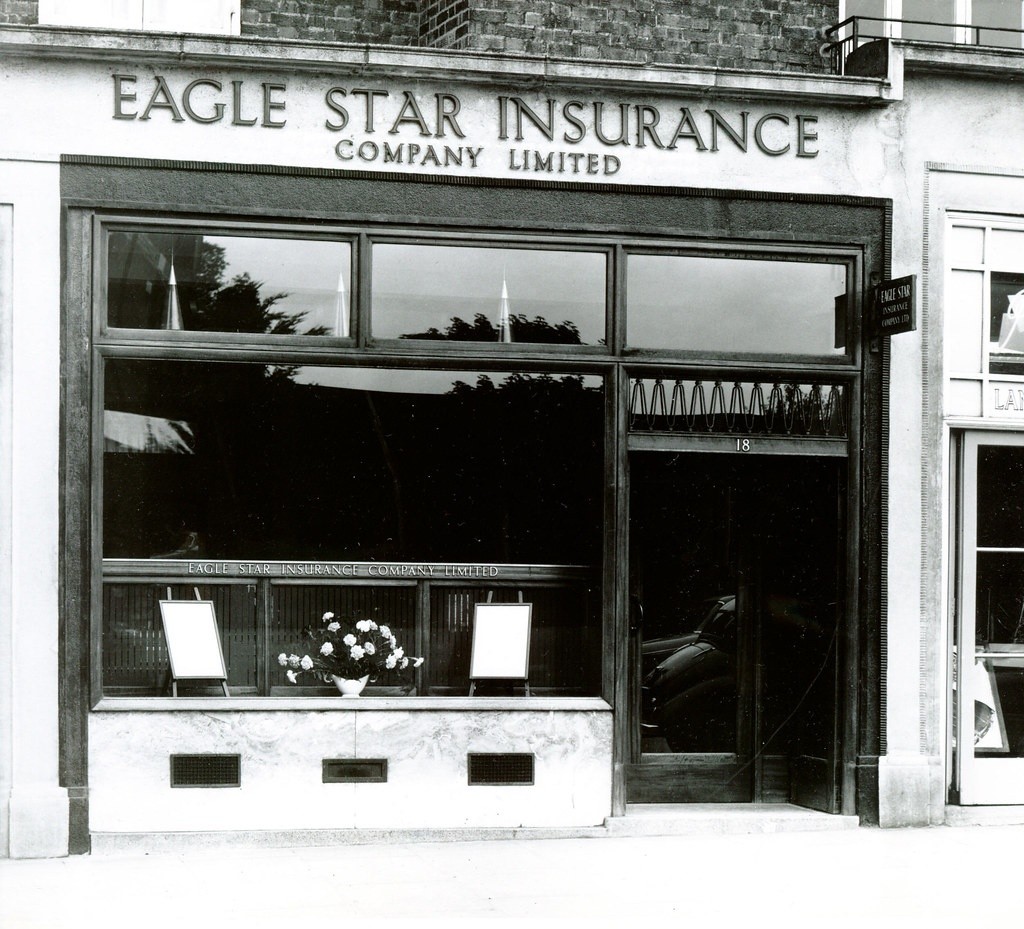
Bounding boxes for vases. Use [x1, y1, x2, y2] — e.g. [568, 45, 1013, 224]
[332, 673, 370, 698]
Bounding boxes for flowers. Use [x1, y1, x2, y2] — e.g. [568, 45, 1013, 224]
[278, 612, 426, 683]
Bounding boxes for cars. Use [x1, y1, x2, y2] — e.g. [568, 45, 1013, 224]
[638, 595, 737, 752]
